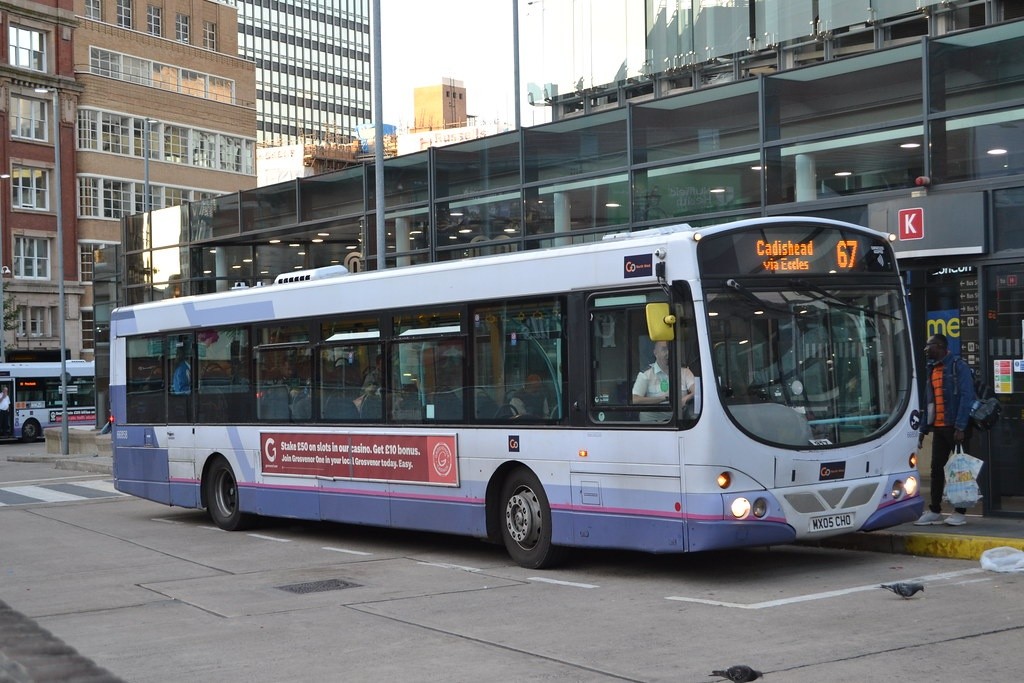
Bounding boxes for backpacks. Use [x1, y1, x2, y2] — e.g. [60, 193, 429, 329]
[952, 358, 1003, 432]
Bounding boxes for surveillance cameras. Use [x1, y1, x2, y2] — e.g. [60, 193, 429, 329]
[5, 270, 11, 274]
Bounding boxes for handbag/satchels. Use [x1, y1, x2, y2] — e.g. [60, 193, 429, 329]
[942, 443, 984, 509]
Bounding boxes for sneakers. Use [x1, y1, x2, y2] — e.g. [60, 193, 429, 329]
[944, 510, 967, 525]
[913, 509, 944, 525]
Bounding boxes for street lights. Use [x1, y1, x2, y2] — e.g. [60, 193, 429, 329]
[34, 87, 69, 455]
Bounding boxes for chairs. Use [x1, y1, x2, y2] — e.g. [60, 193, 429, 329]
[468, 233, 514, 257]
[126, 375, 521, 419]
[345, 250, 365, 275]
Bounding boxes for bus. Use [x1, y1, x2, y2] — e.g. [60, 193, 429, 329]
[108, 215, 924, 571]
[0, 360, 96, 443]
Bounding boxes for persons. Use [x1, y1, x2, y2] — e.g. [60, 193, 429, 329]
[913, 333, 975, 526]
[359, 355, 425, 419]
[0, 387, 11, 436]
[173, 347, 192, 393]
[632, 341, 694, 422]
[509, 374, 550, 421]
